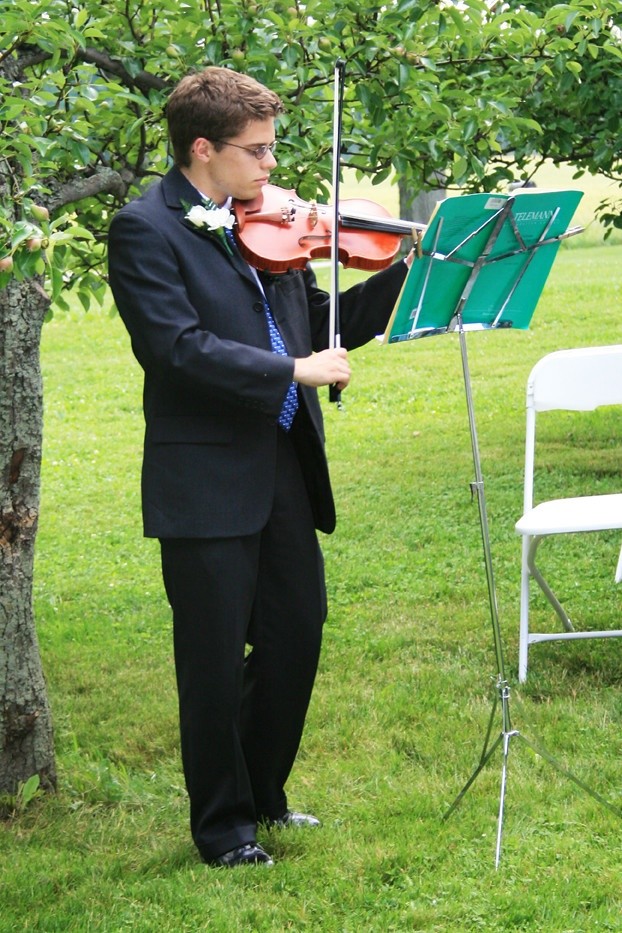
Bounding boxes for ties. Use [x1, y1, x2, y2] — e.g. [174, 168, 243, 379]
[228, 225, 298, 430]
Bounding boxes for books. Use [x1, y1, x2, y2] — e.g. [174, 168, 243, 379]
[380, 186, 586, 343]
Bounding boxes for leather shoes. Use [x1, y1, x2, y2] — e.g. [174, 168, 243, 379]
[216, 843, 273, 868]
[266, 813, 320, 828]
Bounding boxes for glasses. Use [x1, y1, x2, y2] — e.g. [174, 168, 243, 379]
[191, 138, 278, 159]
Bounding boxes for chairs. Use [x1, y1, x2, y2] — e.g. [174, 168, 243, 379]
[516, 347, 621, 685]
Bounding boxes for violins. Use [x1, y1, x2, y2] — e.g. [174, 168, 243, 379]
[233, 179, 438, 272]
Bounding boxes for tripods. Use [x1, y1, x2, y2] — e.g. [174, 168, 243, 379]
[378, 191, 622, 873]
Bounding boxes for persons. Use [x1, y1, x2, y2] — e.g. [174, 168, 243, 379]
[105, 66, 423, 862]
